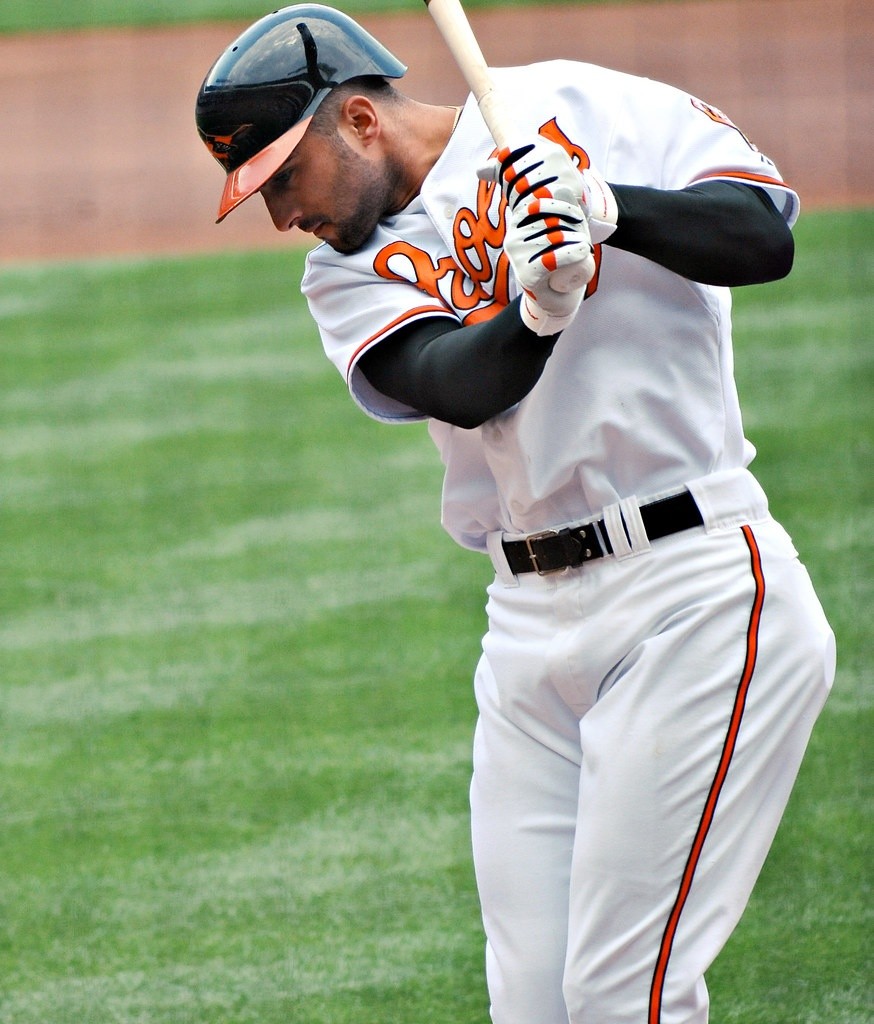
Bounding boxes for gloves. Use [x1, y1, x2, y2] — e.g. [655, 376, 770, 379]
[505, 184, 598, 337]
[502, 132, 619, 247]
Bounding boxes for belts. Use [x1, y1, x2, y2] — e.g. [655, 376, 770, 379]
[501, 488, 703, 575]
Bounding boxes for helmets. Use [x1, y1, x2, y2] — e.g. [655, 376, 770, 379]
[195, 3, 410, 222]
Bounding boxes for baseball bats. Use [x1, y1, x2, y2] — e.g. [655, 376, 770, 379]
[426, 0, 596, 293]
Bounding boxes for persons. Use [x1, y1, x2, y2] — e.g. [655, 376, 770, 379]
[196, 0, 836, 1024]
